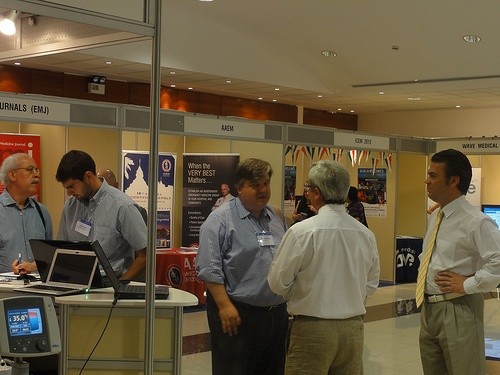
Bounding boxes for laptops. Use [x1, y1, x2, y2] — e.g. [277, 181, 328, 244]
[90, 240, 169, 299]
[12, 248, 99, 297]
[29, 238, 130, 288]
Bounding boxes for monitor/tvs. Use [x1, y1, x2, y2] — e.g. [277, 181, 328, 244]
[481, 204, 500, 230]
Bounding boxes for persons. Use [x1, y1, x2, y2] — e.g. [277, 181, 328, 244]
[195, 158, 288, 375]
[11, 150, 147, 282]
[345, 186, 369, 228]
[211, 184, 235, 212]
[415, 148, 500, 375]
[97, 168, 147, 226]
[290, 188, 318, 227]
[0, 153, 53, 274]
[266, 160, 380, 375]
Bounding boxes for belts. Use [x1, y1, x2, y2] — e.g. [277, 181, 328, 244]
[232, 301, 288, 312]
[423, 292, 465, 303]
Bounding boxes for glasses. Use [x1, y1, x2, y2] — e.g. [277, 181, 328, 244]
[304, 183, 315, 190]
[13, 167, 39, 173]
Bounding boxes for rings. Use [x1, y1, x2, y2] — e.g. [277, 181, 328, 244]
[446, 281, 450, 286]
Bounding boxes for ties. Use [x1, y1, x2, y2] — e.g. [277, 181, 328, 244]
[416, 209, 444, 308]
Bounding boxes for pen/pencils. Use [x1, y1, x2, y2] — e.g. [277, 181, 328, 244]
[19, 253, 21, 275]
